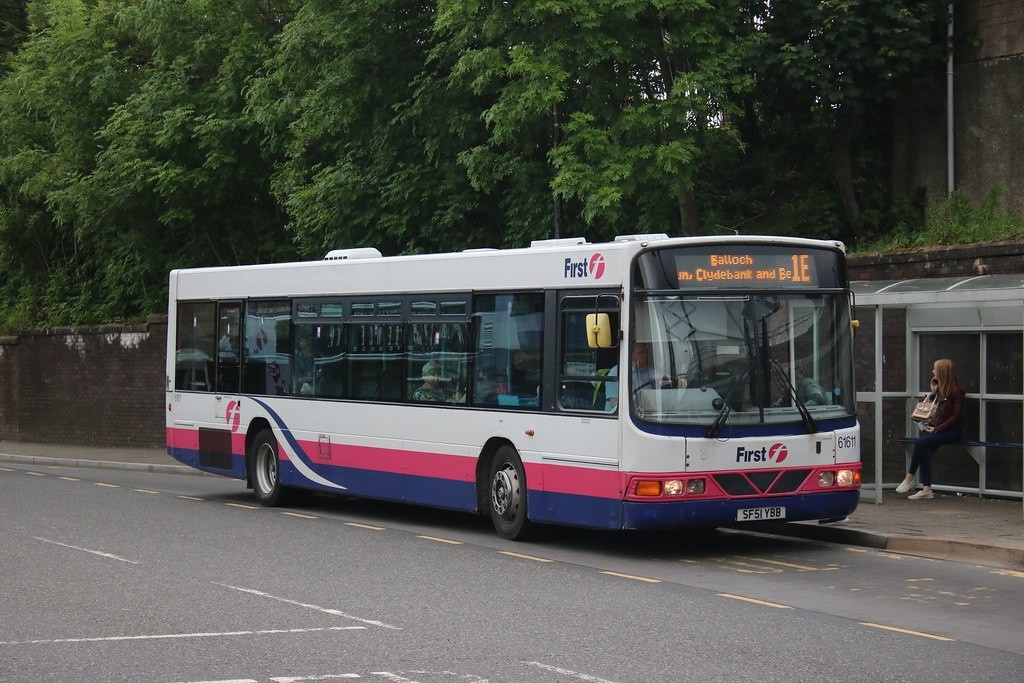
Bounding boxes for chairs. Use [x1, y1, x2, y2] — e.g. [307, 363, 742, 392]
[314, 363, 386, 400]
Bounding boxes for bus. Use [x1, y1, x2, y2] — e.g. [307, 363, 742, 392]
[160, 230, 862, 549]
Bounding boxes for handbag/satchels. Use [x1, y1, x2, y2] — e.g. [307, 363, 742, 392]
[911, 392, 942, 426]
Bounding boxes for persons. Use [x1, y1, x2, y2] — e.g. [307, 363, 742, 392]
[605, 341, 687, 411]
[412, 346, 464, 402]
[295, 339, 327, 396]
[896, 359, 965, 500]
[773, 365, 830, 407]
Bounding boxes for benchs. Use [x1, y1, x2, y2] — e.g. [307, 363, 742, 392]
[896, 437, 1022, 500]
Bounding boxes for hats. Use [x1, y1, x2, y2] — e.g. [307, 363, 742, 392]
[422, 360, 440, 375]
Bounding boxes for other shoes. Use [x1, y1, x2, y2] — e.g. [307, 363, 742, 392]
[908, 490, 933, 499]
[896, 478, 914, 493]
